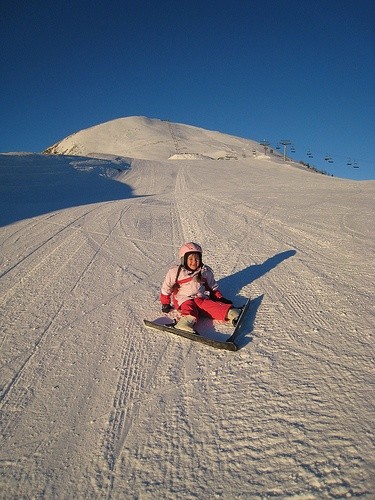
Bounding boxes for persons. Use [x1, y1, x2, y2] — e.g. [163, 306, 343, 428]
[160, 242, 244, 335]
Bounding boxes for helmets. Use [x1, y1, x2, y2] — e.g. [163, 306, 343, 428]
[179, 242, 203, 266]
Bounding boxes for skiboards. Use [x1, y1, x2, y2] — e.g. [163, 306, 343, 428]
[142, 297, 251, 352]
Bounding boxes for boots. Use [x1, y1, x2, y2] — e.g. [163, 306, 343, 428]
[174, 315, 197, 334]
[227, 309, 241, 327]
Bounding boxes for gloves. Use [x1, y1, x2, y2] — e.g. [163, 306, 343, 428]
[219, 297, 232, 305]
[162, 304, 172, 313]
